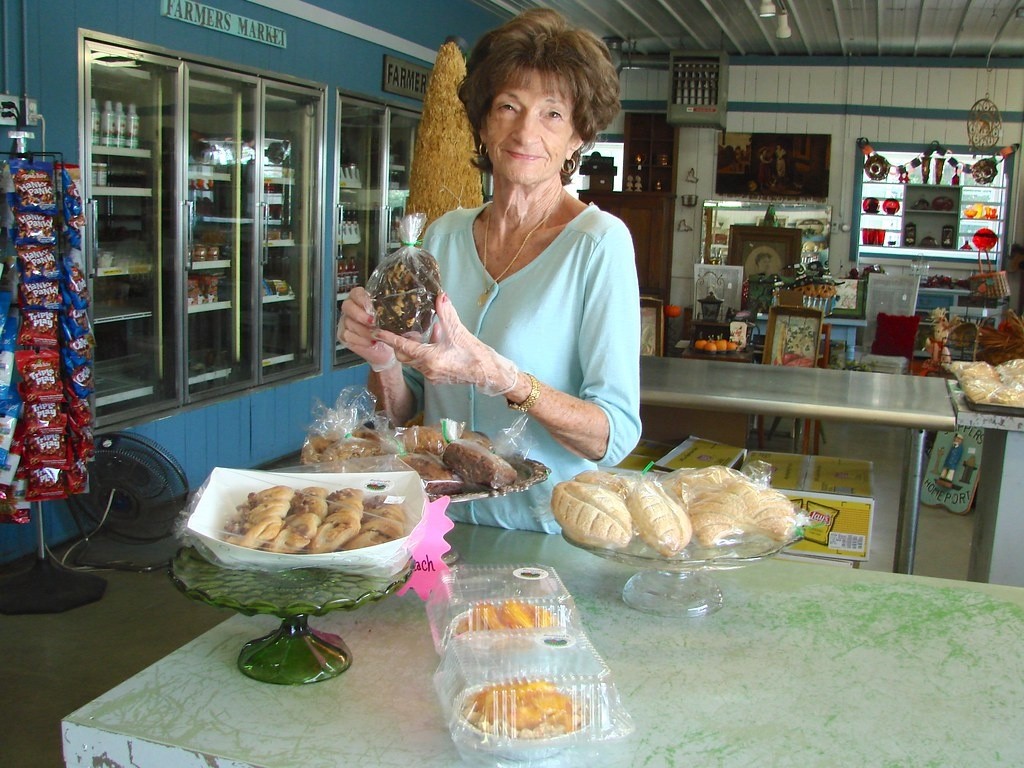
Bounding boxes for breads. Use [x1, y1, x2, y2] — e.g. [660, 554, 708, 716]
[443, 442, 517, 490]
[223, 485, 404, 555]
[394, 453, 467, 496]
[950, 358, 1024, 408]
[550, 465, 795, 558]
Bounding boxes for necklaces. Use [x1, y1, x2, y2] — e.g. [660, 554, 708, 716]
[478, 197, 560, 305]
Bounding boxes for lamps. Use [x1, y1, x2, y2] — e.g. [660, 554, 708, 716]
[775, 13, 792, 38]
[759, 0, 776, 18]
[602, 36, 625, 73]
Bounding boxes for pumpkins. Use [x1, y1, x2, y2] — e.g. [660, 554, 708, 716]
[695, 333, 737, 351]
[664, 304, 681, 317]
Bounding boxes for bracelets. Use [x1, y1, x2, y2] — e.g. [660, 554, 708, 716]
[508, 373, 540, 412]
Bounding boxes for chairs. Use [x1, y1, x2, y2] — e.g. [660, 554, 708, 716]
[838, 272, 923, 375]
[757, 322, 834, 456]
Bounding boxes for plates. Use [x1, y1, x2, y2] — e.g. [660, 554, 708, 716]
[561, 524, 805, 571]
[427, 453, 552, 503]
[167, 544, 416, 616]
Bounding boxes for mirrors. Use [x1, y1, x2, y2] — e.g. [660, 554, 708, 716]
[700, 204, 833, 276]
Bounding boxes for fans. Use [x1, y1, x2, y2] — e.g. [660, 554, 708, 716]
[64, 430, 189, 575]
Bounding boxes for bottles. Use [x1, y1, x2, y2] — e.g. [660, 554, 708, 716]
[338, 202, 361, 244]
[338, 256, 359, 292]
[656, 178, 661, 190]
[92, 163, 107, 186]
[340, 163, 363, 188]
[658, 154, 668, 165]
[188, 273, 218, 303]
[194, 243, 221, 261]
[960, 240, 972, 249]
[770, 275, 782, 306]
[390, 172, 399, 190]
[92, 97, 139, 149]
[952, 167, 959, 184]
[636, 154, 642, 163]
[391, 216, 401, 242]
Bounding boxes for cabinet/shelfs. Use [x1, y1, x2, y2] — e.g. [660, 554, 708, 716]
[860, 181, 1007, 254]
[901, 183, 963, 251]
[622, 110, 679, 194]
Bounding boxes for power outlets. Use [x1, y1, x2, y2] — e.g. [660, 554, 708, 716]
[0, 95, 19, 126]
[25, 97, 39, 127]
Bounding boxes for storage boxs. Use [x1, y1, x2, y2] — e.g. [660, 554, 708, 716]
[760, 305, 824, 368]
[832, 279, 865, 319]
[430, 626, 638, 768]
[424, 562, 583, 659]
[615, 437, 676, 474]
[579, 152, 617, 176]
[641, 296, 664, 357]
[655, 435, 748, 474]
[774, 553, 855, 569]
[712, 230, 729, 245]
[740, 451, 875, 561]
[583, 174, 614, 191]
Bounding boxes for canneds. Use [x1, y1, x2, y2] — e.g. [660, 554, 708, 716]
[91, 163, 107, 187]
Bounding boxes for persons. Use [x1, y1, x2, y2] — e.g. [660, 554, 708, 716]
[336, 8, 643, 533]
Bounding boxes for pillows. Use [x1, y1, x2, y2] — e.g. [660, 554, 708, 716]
[872, 312, 921, 361]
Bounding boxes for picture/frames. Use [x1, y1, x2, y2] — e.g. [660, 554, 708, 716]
[756, 216, 789, 229]
[726, 224, 802, 281]
[693, 263, 744, 320]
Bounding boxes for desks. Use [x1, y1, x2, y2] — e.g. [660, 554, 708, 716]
[61, 519, 1024, 768]
[947, 380, 1024, 587]
[634, 356, 957, 576]
[751, 314, 868, 362]
[919, 288, 972, 308]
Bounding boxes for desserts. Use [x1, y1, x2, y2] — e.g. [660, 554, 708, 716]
[450, 680, 593, 761]
[445, 600, 560, 661]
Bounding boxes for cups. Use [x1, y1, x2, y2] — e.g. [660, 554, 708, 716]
[975, 203, 983, 219]
[920, 157, 946, 184]
[97, 251, 113, 267]
[863, 228, 886, 246]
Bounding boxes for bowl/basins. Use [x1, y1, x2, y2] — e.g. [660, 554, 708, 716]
[964, 210, 977, 218]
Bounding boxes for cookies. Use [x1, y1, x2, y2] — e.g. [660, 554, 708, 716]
[301, 423, 548, 497]
[372, 251, 440, 336]
